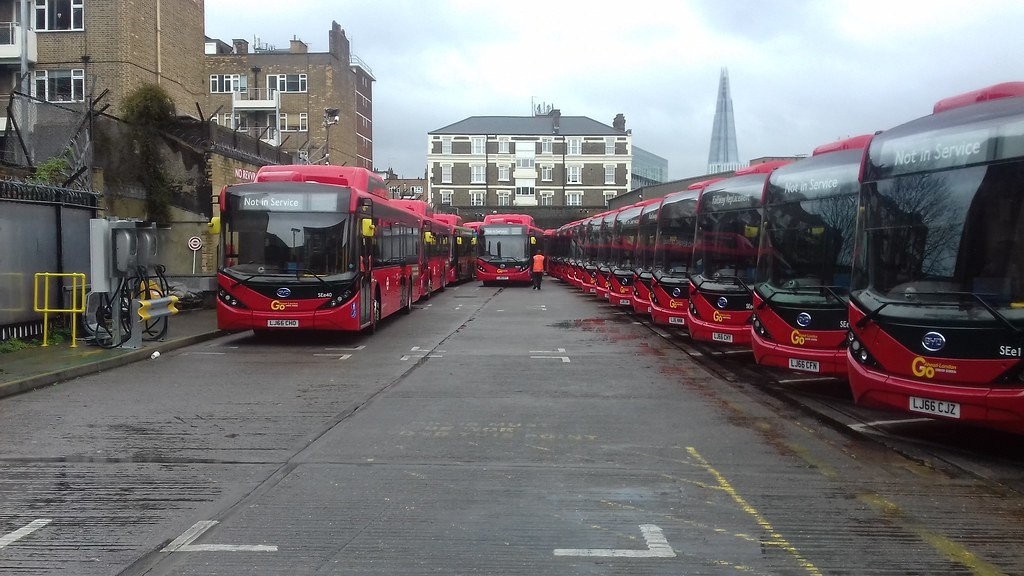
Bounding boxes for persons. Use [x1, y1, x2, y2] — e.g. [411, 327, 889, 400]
[530, 250, 546, 290]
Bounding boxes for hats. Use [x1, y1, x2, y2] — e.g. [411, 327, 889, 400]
[537, 250, 542, 254]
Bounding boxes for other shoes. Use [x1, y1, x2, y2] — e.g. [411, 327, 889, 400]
[538, 288, 541, 289]
[533, 284, 537, 289]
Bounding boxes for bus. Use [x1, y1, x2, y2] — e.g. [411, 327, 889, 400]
[749, 130, 873, 384]
[542, 196, 669, 317]
[648, 177, 736, 330]
[206, 164, 481, 337]
[844, 79, 1024, 440]
[469, 212, 546, 287]
[681, 160, 793, 344]
